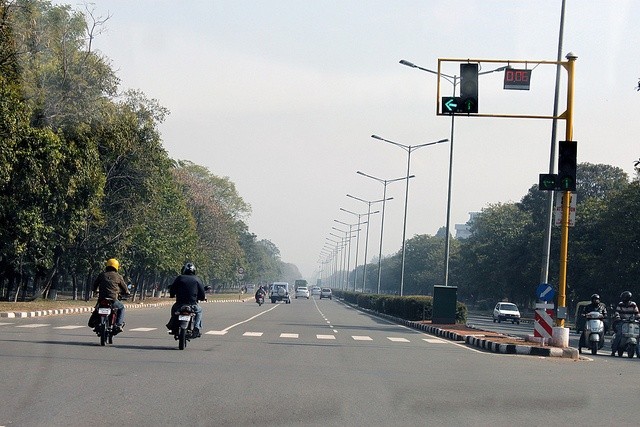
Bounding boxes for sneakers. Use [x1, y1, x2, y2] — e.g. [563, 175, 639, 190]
[115, 325, 124, 332]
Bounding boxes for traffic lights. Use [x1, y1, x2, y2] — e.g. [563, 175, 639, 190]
[504, 68, 531, 90]
[539, 141, 577, 191]
[442, 63, 478, 113]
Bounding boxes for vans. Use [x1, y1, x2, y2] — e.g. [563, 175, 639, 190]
[493, 303, 522, 325]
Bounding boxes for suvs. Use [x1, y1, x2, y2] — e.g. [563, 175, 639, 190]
[270, 282, 290, 304]
[319, 288, 332, 300]
[295, 286, 310, 300]
[311, 285, 321, 295]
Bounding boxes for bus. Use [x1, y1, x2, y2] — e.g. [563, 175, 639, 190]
[295, 279, 308, 292]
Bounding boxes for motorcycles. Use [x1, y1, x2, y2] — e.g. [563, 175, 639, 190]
[167, 283, 213, 351]
[92, 283, 132, 347]
[258, 292, 264, 306]
[610, 315, 640, 359]
[579, 312, 608, 355]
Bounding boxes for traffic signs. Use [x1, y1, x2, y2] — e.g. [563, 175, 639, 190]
[555, 192, 577, 227]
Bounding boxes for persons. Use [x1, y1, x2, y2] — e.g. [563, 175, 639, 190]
[610, 291, 640, 358]
[578, 294, 607, 354]
[255, 287, 266, 303]
[168, 262, 205, 335]
[92, 258, 131, 332]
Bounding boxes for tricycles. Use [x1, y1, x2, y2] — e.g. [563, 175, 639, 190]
[574, 300, 608, 334]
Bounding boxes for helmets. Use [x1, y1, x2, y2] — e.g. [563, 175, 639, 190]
[591, 293, 601, 304]
[106, 258, 120, 273]
[181, 262, 198, 275]
[619, 291, 633, 304]
